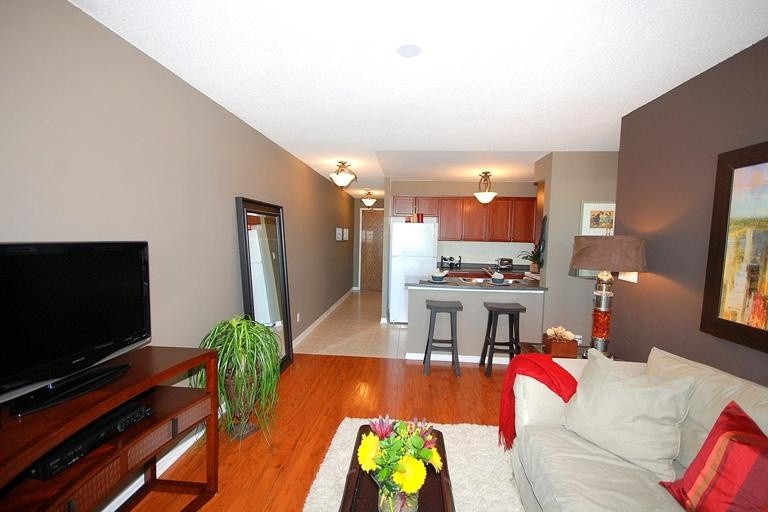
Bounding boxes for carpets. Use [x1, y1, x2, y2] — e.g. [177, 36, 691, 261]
[301, 417, 524, 512]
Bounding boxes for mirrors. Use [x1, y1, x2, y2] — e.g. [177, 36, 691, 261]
[234, 196, 293, 376]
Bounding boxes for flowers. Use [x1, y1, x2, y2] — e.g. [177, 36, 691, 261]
[356, 414, 444, 512]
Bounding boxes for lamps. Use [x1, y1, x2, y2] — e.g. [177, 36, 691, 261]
[569, 234, 649, 361]
[360, 192, 377, 207]
[472, 171, 498, 204]
[328, 160, 356, 186]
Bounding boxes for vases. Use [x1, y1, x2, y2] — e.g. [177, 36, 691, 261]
[377, 489, 418, 512]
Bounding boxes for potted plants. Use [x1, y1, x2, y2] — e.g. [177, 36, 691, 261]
[188, 313, 282, 460]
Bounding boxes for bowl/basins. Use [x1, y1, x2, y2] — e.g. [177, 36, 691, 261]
[432, 275, 444, 281]
[492, 278, 504, 283]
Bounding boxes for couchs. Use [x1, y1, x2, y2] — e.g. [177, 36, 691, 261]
[507, 346, 768, 512]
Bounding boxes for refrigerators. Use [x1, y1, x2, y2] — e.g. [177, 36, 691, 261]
[389, 222, 437, 325]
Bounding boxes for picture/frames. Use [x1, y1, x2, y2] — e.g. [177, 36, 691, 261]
[576, 199, 616, 278]
[699, 139, 768, 354]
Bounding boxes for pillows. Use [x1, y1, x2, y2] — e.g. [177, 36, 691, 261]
[657, 400, 768, 512]
[558, 348, 704, 481]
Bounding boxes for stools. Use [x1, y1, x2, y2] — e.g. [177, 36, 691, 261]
[422, 300, 462, 376]
[479, 302, 526, 377]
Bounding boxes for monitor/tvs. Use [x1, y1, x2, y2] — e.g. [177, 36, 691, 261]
[0, 240, 153, 418]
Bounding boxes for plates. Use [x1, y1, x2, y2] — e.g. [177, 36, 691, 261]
[430, 280, 446, 284]
[490, 282, 509, 286]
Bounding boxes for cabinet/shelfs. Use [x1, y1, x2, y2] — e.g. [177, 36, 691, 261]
[392, 196, 439, 216]
[0, 346, 218, 512]
[438, 196, 537, 242]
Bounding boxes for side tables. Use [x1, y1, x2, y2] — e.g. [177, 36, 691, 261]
[517, 343, 611, 360]
